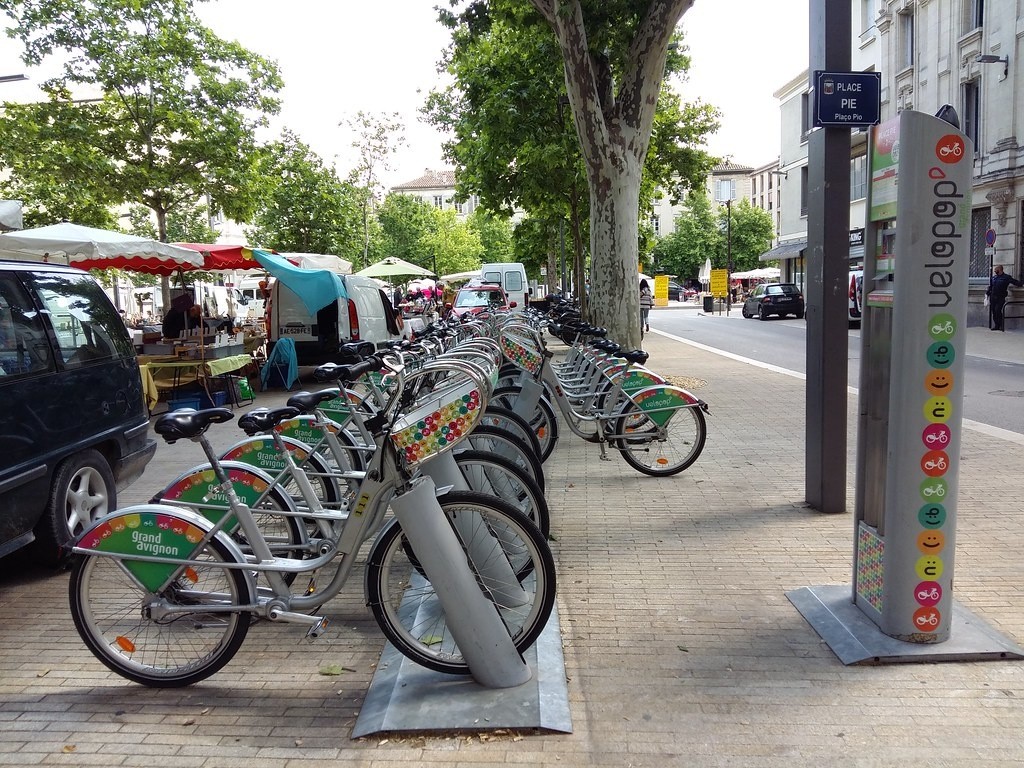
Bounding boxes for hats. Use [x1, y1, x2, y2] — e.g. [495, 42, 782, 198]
[436, 281, 441, 288]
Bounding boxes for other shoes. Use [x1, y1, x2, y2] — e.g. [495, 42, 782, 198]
[991, 328, 999, 330]
[646, 323, 649, 331]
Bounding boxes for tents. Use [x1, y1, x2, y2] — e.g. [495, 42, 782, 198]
[441, 269, 482, 283]
[0, 221, 354, 373]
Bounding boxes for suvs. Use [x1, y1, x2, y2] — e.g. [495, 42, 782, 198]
[0, 258, 158, 576]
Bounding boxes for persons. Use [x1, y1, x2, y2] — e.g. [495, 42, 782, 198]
[731, 281, 752, 304]
[394, 281, 457, 313]
[162, 303, 210, 339]
[986, 264, 1022, 331]
[584, 279, 589, 296]
[640, 279, 652, 332]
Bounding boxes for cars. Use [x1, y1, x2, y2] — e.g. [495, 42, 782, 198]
[668, 280, 701, 301]
[445, 282, 517, 324]
[742, 282, 805, 321]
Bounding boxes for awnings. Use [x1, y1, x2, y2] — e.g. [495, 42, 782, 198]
[759, 242, 807, 262]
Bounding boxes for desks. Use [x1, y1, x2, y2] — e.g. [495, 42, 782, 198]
[139, 354, 253, 419]
[241, 334, 267, 381]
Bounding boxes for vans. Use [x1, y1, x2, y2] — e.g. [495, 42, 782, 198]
[266, 270, 413, 366]
[480, 261, 533, 312]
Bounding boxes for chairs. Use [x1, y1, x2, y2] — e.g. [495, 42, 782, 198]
[260, 338, 303, 392]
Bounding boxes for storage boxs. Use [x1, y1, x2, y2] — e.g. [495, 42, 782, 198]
[142, 343, 246, 359]
[167, 397, 201, 413]
[168, 389, 200, 399]
[193, 391, 225, 408]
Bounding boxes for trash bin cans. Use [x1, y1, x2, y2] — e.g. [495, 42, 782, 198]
[703, 295, 712, 312]
[679, 292, 684, 302]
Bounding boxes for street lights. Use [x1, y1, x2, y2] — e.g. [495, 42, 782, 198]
[722, 200, 732, 311]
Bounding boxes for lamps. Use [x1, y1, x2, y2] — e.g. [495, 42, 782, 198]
[771, 171, 787, 179]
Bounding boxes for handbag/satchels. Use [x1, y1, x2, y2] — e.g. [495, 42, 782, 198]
[650, 297, 655, 306]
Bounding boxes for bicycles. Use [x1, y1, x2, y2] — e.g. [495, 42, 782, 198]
[128, 290, 712, 620]
[56, 339, 557, 689]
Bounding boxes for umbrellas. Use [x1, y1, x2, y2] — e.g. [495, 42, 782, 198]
[354, 256, 437, 308]
[639, 273, 651, 279]
[698, 257, 711, 293]
[729, 266, 780, 288]
